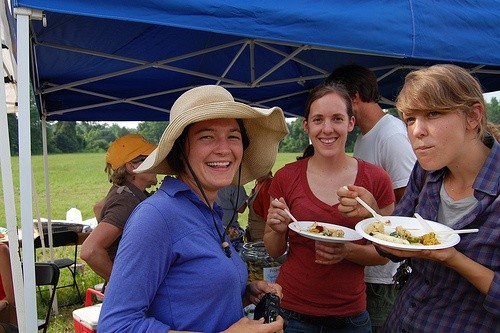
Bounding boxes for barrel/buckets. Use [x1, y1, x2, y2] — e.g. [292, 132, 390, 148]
[94, 283, 104, 301]
[242, 242, 287, 283]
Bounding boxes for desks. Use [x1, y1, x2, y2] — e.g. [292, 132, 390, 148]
[0, 225, 92, 249]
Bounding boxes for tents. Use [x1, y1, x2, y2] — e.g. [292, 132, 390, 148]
[1, 0, 500, 331]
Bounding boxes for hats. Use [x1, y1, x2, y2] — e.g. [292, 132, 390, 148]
[106, 133, 157, 171]
[133, 85, 290, 186]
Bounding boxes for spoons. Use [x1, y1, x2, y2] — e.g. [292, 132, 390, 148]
[343, 186, 391, 225]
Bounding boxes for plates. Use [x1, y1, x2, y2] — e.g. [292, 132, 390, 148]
[355, 216, 461, 251]
[288, 221, 363, 243]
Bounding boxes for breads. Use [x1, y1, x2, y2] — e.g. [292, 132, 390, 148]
[364, 222, 384, 235]
[373, 233, 410, 245]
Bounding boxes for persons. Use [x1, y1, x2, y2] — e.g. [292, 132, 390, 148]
[0, 241, 19, 333]
[338, 63, 500, 333]
[80, 134, 159, 296]
[329, 59, 417, 333]
[95, 85, 290, 333]
[214, 184, 249, 232]
[263, 84, 395, 333]
[248, 169, 275, 242]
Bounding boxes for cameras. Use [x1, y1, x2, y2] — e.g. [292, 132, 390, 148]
[254, 293, 282, 323]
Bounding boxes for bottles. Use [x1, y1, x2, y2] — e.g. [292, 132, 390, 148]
[66, 206, 82, 224]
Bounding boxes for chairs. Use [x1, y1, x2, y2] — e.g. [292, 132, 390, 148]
[7, 232, 83, 333]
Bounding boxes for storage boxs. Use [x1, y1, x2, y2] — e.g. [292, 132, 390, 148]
[94, 283, 104, 302]
[72, 288, 105, 333]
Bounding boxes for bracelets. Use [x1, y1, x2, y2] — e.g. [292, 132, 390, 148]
[2, 299, 11, 313]
